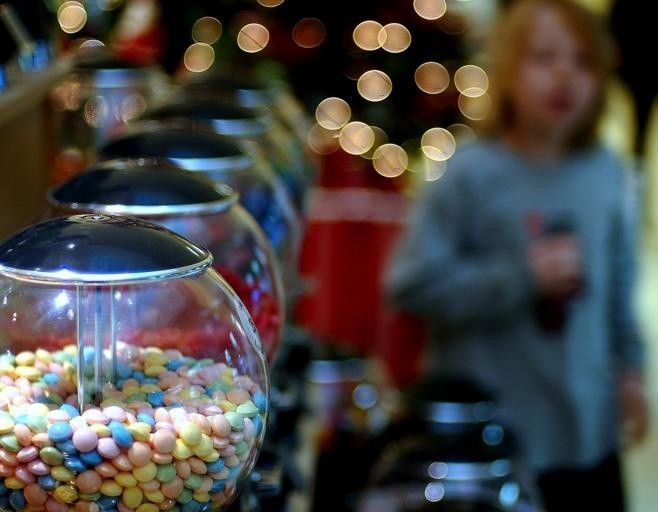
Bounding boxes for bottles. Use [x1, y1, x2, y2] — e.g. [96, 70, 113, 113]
[45, 71, 323, 368]
[0, 209, 269, 512]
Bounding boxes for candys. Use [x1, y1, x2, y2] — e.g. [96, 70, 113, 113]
[0, 264, 281, 512]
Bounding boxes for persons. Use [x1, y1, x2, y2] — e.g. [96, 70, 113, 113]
[382, 0, 649, 512]
